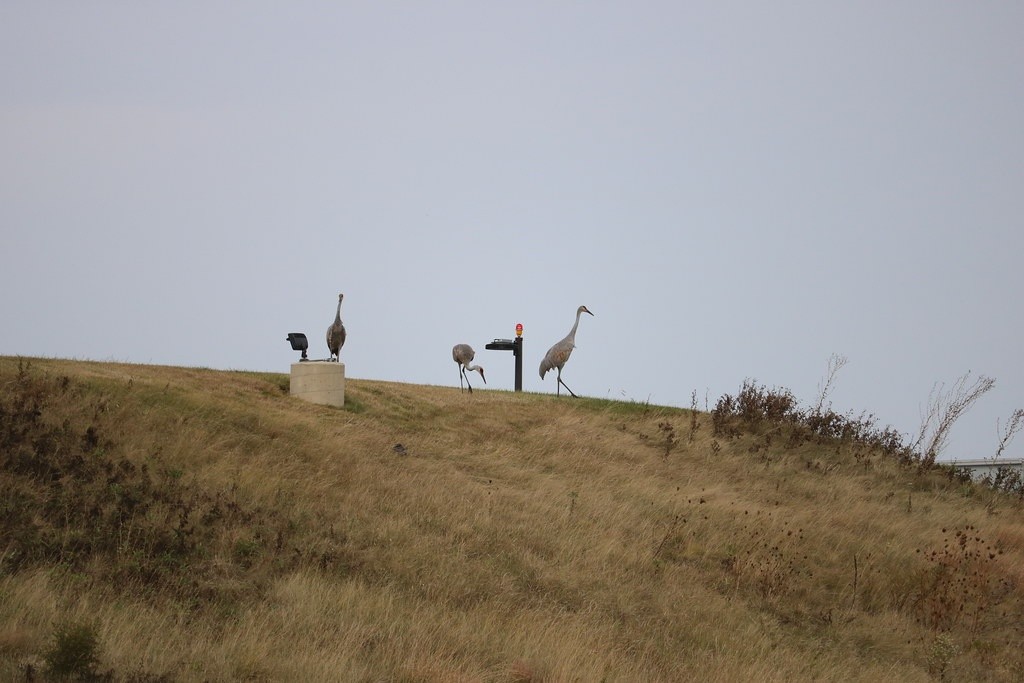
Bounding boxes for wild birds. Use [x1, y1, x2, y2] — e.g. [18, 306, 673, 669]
[539, 305, 594, 398]
[452, 343, 487, 391]
[325, 293, 347, 363]
